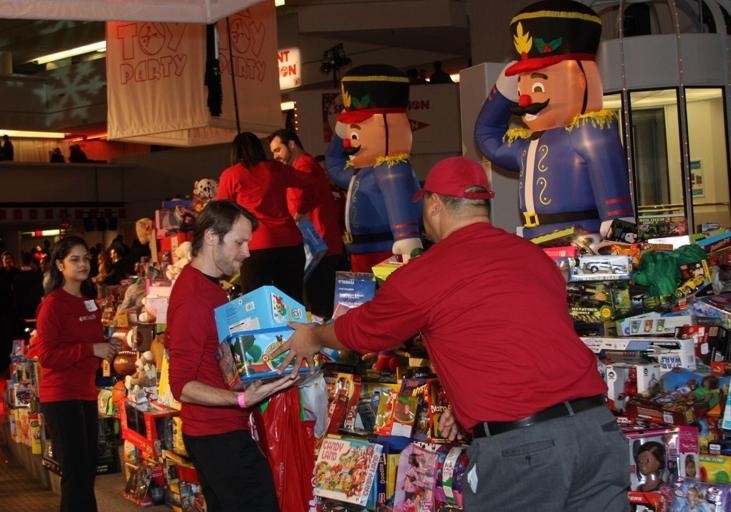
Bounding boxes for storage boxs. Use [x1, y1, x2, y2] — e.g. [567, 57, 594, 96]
[214, 321, 323, 392]
[212, 285, 306, 347]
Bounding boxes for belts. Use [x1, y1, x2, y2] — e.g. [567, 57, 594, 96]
[519, 206, 601, 228]
[472, 392, 610, 439]
[342, 230, 394, 247]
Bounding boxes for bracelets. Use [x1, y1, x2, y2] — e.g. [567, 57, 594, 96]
[237, 390, 250, 409]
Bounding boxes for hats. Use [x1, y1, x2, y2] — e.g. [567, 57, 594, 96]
[334, 63, 412, 123]
[413, 156, 497, 203]
[505, 0, 603, 78]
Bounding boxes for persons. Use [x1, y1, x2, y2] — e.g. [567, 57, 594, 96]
[202, 130, 322, 306]
[400, 451, 422, 503]
[0, 135, 15, 163]
[0, 232, 151, 383]
[268, 154, 636, 511]
[33, 233, 126, 512]
[74, 143, 88, 162]
[473, 1, 640, 245]
[49, 146, 66, 163]
[160, 197, 303, 512]
[323, 63, 429, 277]
[266, 127, 344, 318]
[69, 145, 83, 163]
[631, 375, 730, 509]
